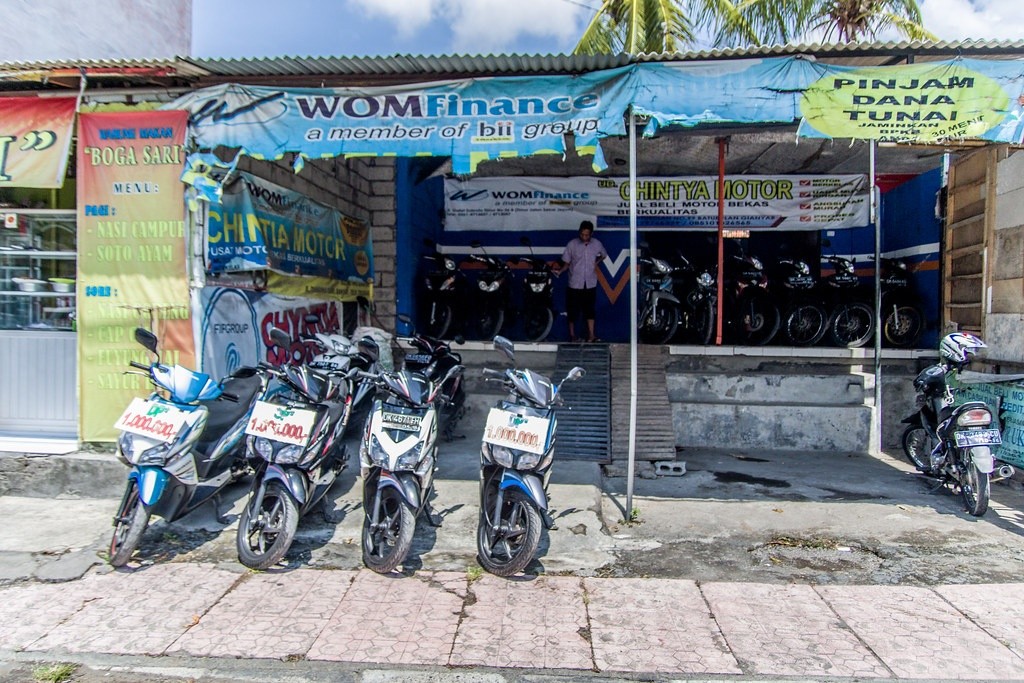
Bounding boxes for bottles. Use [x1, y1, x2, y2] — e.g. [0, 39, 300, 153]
[72, 317, 76, 332]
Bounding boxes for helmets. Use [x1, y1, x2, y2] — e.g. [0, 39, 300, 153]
[939, 332, 987, 363]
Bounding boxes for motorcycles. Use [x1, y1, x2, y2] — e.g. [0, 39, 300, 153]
[900, 360, 1015, 516]
[416, 236, 925, 347]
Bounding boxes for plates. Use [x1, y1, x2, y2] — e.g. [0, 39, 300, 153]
[48, 277, 75, 283]
[12, 278, 48, 284]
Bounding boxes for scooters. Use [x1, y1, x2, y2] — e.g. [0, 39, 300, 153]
[297, 295, 469, 444]
[356, 339, 465, 574]
[108, 327, 265, 567]
[476, 335, 588, 577]
[236, 327, 364, 569]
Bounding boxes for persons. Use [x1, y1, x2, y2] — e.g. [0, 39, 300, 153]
[551, 220, 608, 343]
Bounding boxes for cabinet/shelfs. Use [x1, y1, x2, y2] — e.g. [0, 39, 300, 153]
[0, 208, 77, 333]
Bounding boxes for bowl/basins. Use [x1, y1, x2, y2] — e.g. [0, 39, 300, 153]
[49, 282, 74, 292]
[18, 282, 50, 292]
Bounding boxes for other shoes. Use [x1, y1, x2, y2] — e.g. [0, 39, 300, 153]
[570, 337, 586, 342]
[588, 337, 602, 343]
